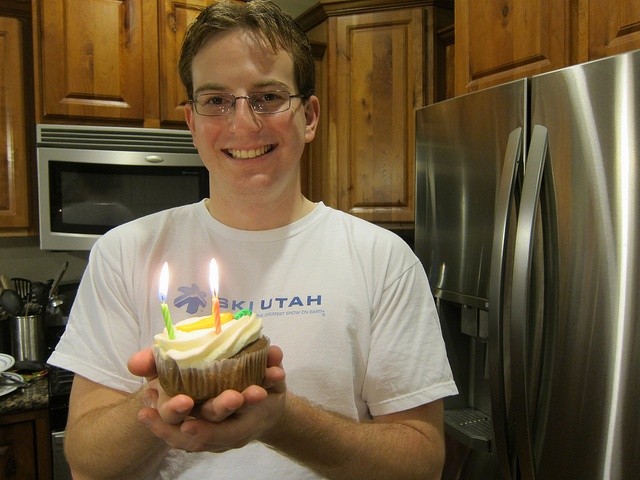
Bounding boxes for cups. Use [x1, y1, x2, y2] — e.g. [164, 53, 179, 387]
[10, 316, 47, 370]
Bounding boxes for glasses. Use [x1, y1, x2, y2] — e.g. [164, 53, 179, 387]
[188, 89, 311, 117]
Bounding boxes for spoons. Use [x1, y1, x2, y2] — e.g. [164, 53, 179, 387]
[1, 289, 24, 315]
[49, 298, 64, 308]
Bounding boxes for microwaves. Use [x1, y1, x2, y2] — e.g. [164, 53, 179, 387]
[35, 122, 211, 251]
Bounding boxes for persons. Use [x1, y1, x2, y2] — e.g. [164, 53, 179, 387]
[43, 1, 460, 480]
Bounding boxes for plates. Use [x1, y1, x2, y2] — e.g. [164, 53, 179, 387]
[0, 352, 15, 372]
[0, 372, 24, 396]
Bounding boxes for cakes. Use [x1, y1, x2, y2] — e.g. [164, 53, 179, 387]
[150, 309, 270, 401]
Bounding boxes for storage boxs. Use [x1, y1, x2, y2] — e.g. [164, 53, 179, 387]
[1, 17, 29, 237]
[334, 6, 431, 229]
[452, 0, 640, 97]
[36, 1, 216, 129]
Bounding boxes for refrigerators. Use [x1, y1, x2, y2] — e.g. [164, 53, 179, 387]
[415, 49, 640, 479]
